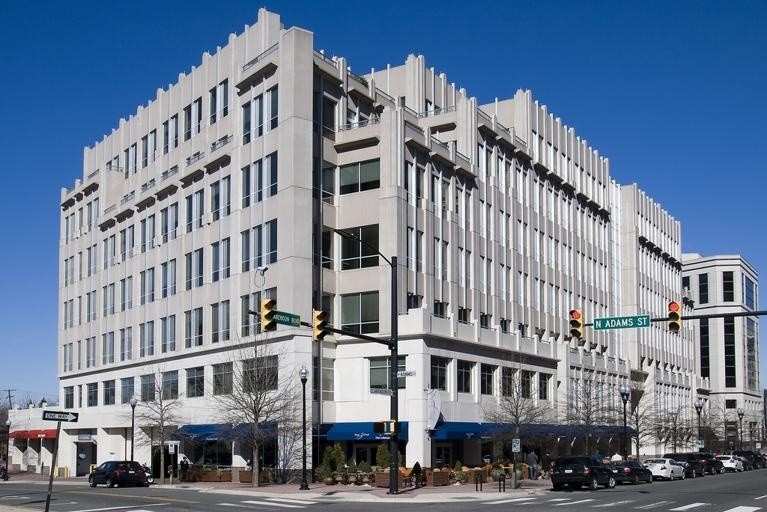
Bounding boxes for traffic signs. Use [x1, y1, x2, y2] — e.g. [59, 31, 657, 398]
[593, 316, 651, 330]
[42, 409, 78, 422]
[276, 312, 301, 328]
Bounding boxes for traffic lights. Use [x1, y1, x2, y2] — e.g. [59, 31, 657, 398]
[569, 310, 583, 339]
[374, 423, 387, 434]
[312, 307, 328, 342]
[668, 301, 681, 333]
[389, 421, 400, 433]
[259, 297, 277, 332]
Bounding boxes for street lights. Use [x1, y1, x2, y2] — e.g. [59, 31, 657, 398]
[3, 419, 11, 480]
[129, 395, 136, 461]
[617, 383, 631, 459]
[299, 364, 308, 489]
[694, 400, 703, 451]
[736, 408, 744, 450]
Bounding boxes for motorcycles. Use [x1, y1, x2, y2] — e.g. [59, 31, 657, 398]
[141, 462, 154, 484]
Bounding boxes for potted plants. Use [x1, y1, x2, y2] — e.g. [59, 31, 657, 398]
[314, 442, 372, 487]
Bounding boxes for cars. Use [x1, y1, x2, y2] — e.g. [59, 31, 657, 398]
[664, 455, 705, 478]
[88, 461, 146, 488]
[607, 459, 653, 483]
[733, 450, 759, 470]
[702, 453, 725, 475]
[550, 454, 616, 490]
[642, 457, 685, 480]
[715, 454, 744, 472]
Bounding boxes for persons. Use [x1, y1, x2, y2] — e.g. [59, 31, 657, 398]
[179, 457, 189, 482]
[541, 451, 553, 479]
[527, 449, 539, 480]
[591, 450, 602, 462]
[611, 452, 622, 461]
